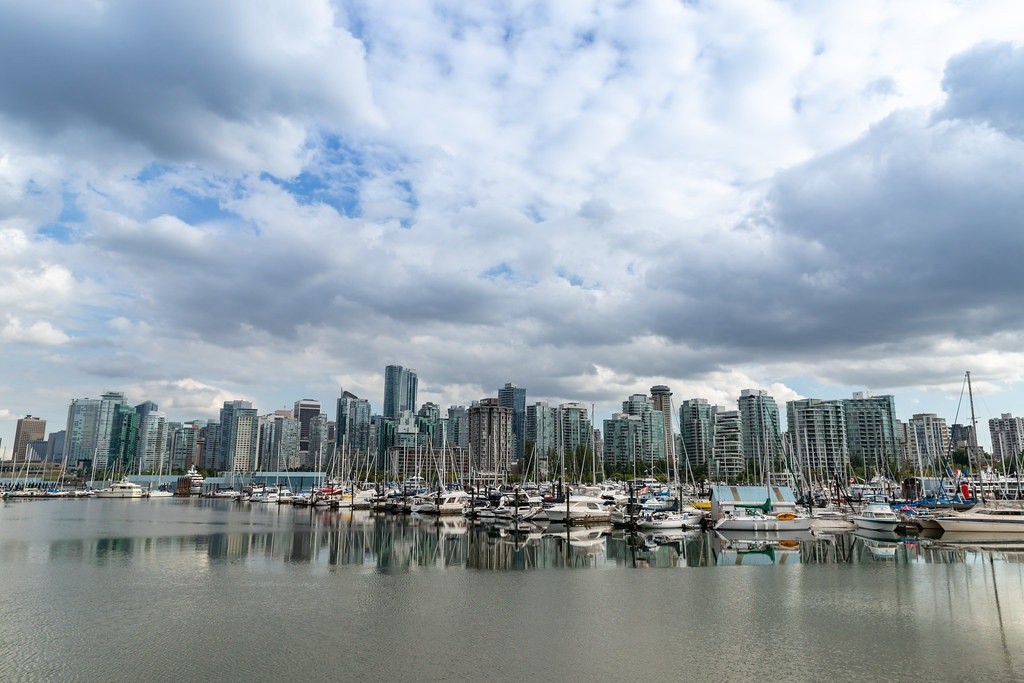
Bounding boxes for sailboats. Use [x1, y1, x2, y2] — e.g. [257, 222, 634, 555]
[0, 369, 1024, 546]
